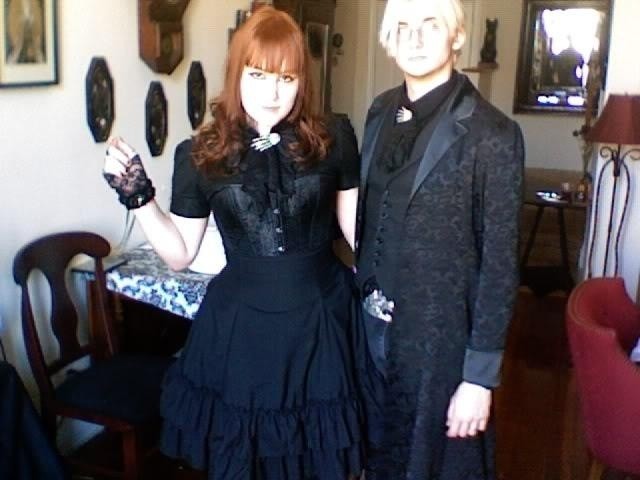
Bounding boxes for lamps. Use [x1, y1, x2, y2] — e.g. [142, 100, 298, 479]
[585, 93, 640, 279]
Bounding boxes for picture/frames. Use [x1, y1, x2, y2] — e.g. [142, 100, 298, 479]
[512, 0, 614, 119]
[0, 0, 59, 88]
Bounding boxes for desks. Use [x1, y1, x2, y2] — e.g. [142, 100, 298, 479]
[77, 240, 217, 323]
[513, 185, 586, 284]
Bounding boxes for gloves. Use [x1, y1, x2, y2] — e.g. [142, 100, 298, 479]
[101, 146, 156, 209]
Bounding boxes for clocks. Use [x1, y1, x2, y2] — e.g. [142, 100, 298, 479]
[138, 1, 193, 75]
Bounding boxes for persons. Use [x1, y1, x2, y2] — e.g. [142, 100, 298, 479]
[100, 6, 362, 480]
[349, 0, 525, 478]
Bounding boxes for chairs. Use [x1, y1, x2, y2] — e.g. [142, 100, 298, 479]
[9, 231, 164, 479]
[568, 278, 640, 480]
